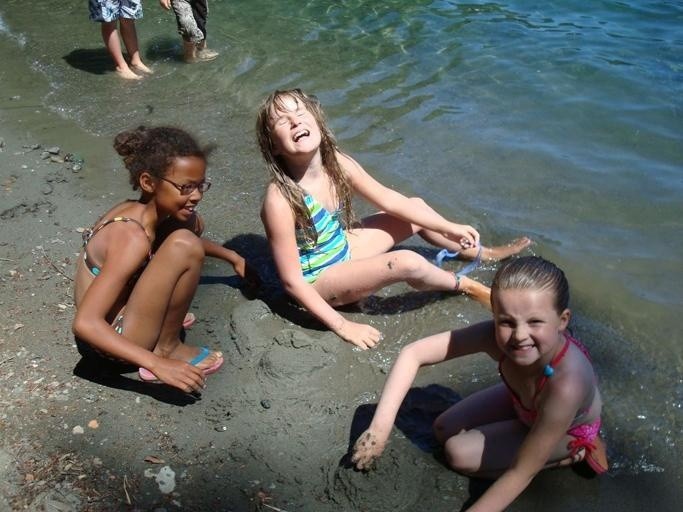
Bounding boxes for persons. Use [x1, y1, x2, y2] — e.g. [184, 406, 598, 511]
[255, 87, 531, 352]
[89, 0, 151, 80]
[350, 257, 607, 512]
[71, 125, 262, 394]
[160, 0, 210, 61]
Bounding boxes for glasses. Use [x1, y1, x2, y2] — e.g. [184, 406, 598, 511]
[153, 173, 212, 196]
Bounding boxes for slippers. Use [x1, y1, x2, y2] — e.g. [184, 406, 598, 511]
[180, 311, 196, 329]
[137, 346, 224, 383]
